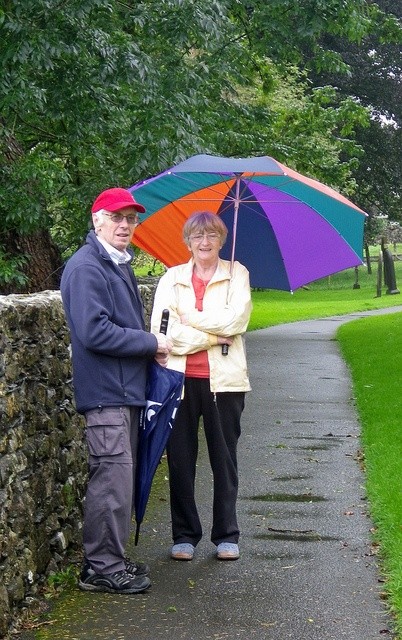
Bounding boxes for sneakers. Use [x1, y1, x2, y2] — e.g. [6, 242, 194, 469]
[78, 563, 151, 593]
[172, 543, 194, 560]
[217, 543, 240, 560]
[123, 558, 150, 577]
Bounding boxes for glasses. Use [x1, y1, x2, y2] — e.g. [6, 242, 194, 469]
[189, 232, 222, 241]
[101, 213, 141, 224]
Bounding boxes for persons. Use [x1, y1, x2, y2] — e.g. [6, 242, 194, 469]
[149, 210, 254, 561]
[59, 187, 174, 595]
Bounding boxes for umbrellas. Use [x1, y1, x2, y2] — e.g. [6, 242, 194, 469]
[114, 153, 369, 355]
[134, 310, 187, 546]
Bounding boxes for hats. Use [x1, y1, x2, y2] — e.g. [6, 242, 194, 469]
[92, 188, 146, 213]
